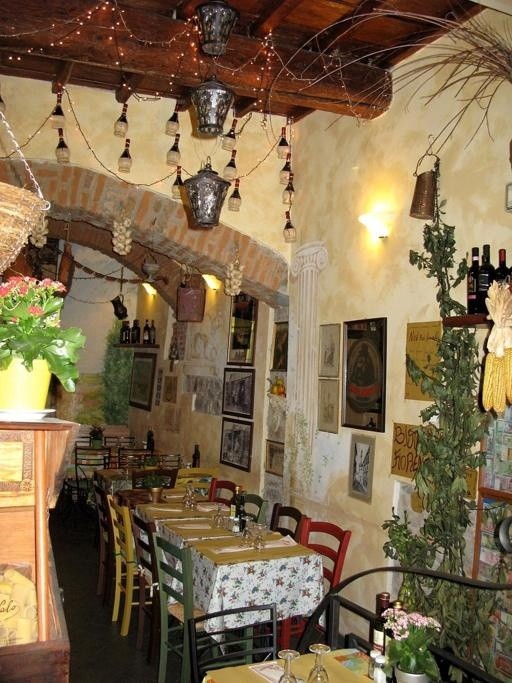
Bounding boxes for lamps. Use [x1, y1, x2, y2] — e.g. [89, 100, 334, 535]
[142, 282, 157, 295]
[201, 274, 222, 290]
[358, 213, 393, 239]
[183, 0, 238, 229]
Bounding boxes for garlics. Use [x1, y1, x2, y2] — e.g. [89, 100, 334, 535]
[112, 217, 132, 256]
[224, 263, 243, 296]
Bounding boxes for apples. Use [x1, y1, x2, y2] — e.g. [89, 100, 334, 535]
[272, 379, 286, 397]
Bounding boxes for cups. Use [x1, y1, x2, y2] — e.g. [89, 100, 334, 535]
[150, 486, 163, 505]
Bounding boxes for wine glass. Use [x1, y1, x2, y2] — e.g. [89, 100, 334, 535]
[142, 263, 161, 283]
[277, 649, 300, 683]
[239, 515, 253, 550]
[307, 644, 332, 683]
[253, 523, 265, 554]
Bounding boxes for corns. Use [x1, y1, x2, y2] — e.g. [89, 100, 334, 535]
[491, 357, 506, 415]
[503, 348, 512, 405]
[481, 353, 498, 412]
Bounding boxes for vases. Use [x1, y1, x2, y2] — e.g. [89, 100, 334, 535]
[394, 665, 432, 683]
[0, 359, 56, 410]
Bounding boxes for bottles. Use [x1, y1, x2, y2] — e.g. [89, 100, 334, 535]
[145, 425, 155, 451]
[213, 506, 223, 528]
[372, 591, 390, 658]
[467, 244, 512, 316]
[367, 650, 383, 680]
[374, 654, 388, 683]
[192, 444, 201, 468]
[182, 489, 191, 510]
[226, 484, 247, 536]
[120, 319, 157, 345]
[383, 599, 407, 683]
[189, 492, 198, 513]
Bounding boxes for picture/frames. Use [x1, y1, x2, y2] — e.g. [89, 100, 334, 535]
[270, 321, 288, 373]
[317, 380, 340, 435]
[341, 317, 387, 434]
[226, 293, 259, 367]
[221, 367, 255, 419]
[465, 492, 512, 679]
[159, 371, 184, 408]
[219, 416, 257, 473]
[318, 323, 340, 378]
[478, 406, 512, 498]
[127, 351, 159, 412]
[348, 433, 375, 506]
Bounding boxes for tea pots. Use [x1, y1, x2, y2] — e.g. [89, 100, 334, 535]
[110, 294, 128, 320]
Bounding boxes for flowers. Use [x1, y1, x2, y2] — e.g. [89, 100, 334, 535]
[0, 275, 86, 392]
[88, 423, 105, 440]
[380, 608, 442, 679]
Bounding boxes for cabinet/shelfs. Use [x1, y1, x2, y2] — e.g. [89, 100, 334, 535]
[0, 418, 70, 683]
